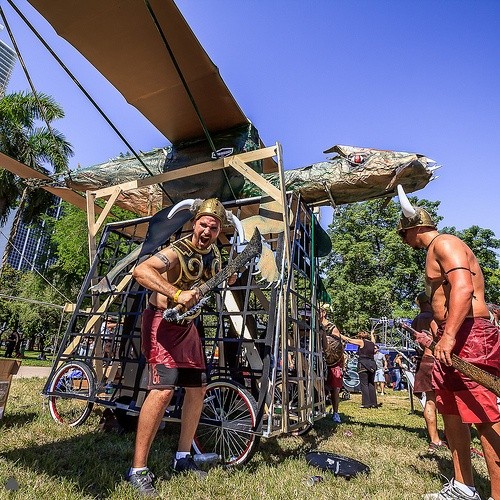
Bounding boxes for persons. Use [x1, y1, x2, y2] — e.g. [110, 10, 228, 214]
[260, 299, 344, 424]
[396, 182, 500, 500]
[38, 336, 47, 360]
[340, 329, 377, 409]
[80, 316, 123, 368]
[374, 345, 388, 396]
[212, 260, 259, 403]
[383, 352, 416, 391]
[125, 198, 238, 496]
[410, 291, 500, 461]
[5, 330, 22, 358]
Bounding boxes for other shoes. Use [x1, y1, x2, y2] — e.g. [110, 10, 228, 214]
[381, 393, 384, 396]
[333, 412, 341, 422]
[428, 440, 449, 454]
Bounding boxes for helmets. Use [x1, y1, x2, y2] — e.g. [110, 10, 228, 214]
[395, 185, 437, 233]
[167, 198, 245, 245]
[317, 301, 333, 313]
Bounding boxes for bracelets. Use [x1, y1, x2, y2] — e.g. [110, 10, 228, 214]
[174, 289, 182, 304]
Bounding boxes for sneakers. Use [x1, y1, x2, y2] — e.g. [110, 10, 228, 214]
[165, 454, 209, 480]
[421, 474, 482, 500]
[124, 466, 162, 500]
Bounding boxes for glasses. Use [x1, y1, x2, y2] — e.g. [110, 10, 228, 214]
[398, 229, 407, 239]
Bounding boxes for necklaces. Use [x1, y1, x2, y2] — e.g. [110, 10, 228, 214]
[425, 234, 440, 251]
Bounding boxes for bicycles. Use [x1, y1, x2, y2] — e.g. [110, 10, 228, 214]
[46, 351, 316, 471]
[342, 367, 360, 388]
